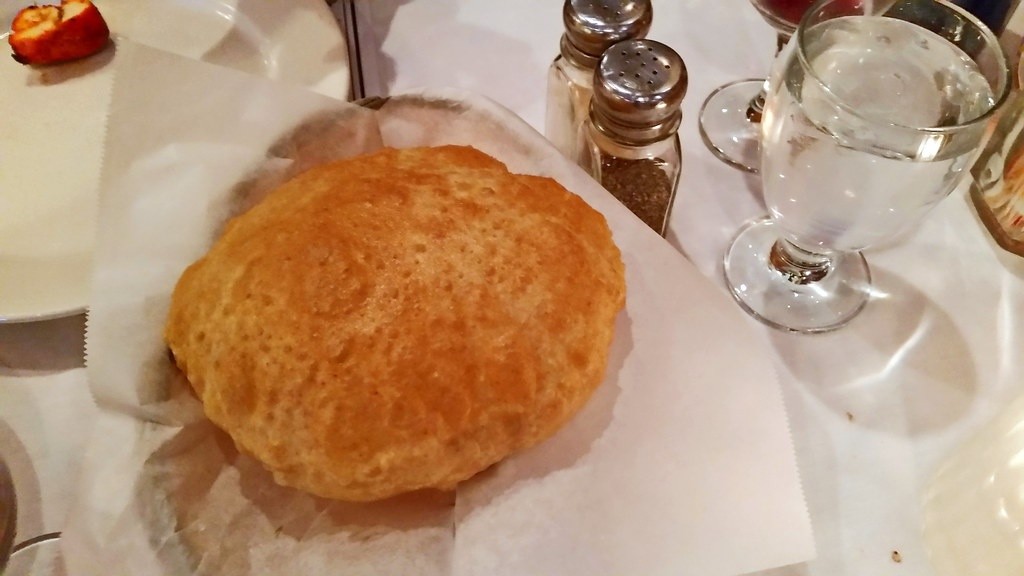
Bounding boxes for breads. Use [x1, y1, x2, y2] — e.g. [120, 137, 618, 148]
[163, 144, 623, 504]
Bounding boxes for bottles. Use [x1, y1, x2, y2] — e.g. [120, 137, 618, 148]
[574, 40, 689, 239]
[548, 1, 653, 171]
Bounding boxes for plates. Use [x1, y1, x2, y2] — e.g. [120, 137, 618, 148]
[1, 1, 350, 324]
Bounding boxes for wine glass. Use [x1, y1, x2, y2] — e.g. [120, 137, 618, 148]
[698, 1, 904, 174]
[724, 1, 1014, 335]
